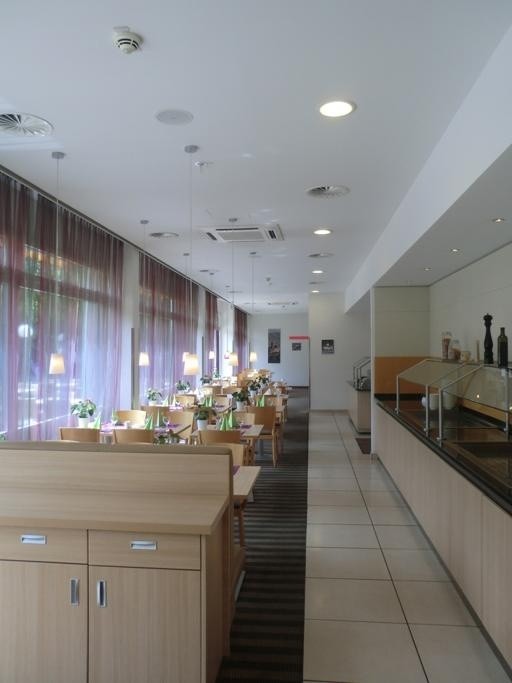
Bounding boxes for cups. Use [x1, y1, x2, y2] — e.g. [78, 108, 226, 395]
[124, 421, 131, 429]
[460, 350, 471, 360]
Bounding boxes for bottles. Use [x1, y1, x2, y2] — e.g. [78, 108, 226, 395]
[497, 327, 508, 368]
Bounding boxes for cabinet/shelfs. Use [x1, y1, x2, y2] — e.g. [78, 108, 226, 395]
[2, 450, 232, 681]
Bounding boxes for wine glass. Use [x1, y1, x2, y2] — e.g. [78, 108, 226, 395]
[163, 416, 170, 430]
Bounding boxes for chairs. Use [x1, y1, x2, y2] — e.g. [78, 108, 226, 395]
[57, 366, 289, 551]
